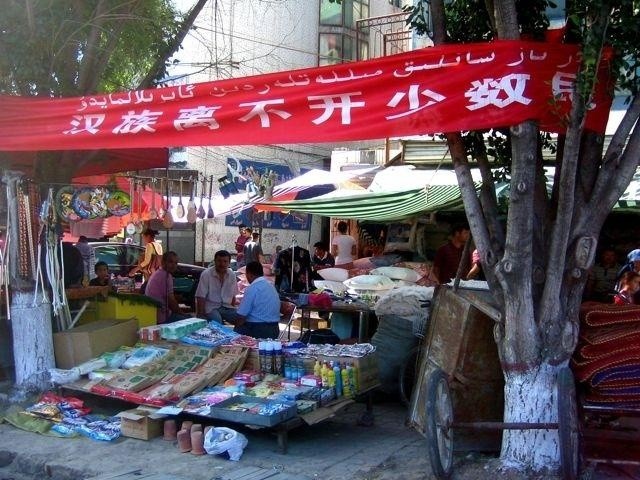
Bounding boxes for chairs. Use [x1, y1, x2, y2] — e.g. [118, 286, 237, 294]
[278, 301, 297, 342]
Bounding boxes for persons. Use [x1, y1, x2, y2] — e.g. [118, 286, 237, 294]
[433, 223, 503, 285]
[270, 246, 284, 262]
[244, 234, 264, 262]
[235, 223, 248, 262]
[236, 227, 255, 265]
[331, 221, 357, 270]
[194, 249, 241, 327]
[235, 259, 281, 338]
[146, 251, 188, 322]
[309, 240, 335, 271]
[591, 241, 640, 305]
[128, 229, 163, 290]
[91, 262, 110, 288]
[75, 234, 96, 285]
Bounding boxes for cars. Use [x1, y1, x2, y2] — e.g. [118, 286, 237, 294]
[73, 240, 208, 313]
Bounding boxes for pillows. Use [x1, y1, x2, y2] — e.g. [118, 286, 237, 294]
[236, 254, 437, 309]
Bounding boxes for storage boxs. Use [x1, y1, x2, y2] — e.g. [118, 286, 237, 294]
[315, 351, 380, 394]
[114, 404, 169, 441]
[53, 319, 138, 370]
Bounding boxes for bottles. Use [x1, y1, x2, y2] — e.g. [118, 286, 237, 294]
[313, 359, 359, 399]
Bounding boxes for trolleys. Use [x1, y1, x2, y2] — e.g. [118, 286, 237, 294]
[403, 230, 560, 480]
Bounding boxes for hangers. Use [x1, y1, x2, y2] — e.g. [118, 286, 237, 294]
[288, 240, 298, 248]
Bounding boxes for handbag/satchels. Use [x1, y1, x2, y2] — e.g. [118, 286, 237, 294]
[137, 243, 164, 274]
[309, 291, 333, 310]
